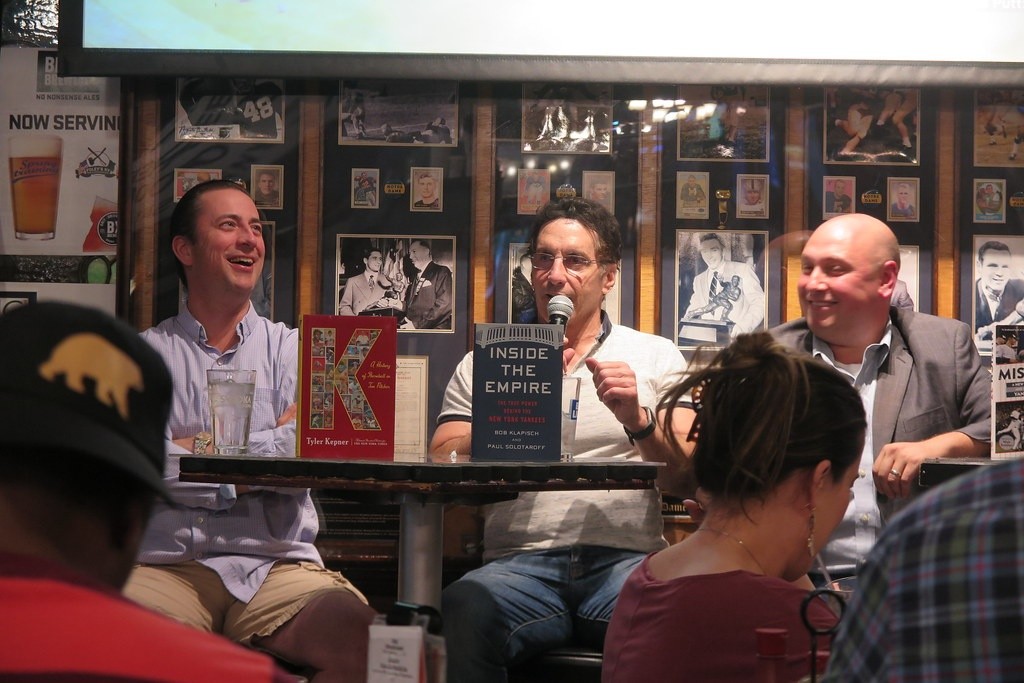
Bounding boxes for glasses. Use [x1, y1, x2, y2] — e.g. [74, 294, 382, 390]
[531, 253, 621, 271]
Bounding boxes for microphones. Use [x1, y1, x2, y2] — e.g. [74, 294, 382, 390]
[547, 295, 574, 335]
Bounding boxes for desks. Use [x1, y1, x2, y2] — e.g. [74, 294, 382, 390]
[918, 456, 1005, 489]
[180, 453, 668, 636]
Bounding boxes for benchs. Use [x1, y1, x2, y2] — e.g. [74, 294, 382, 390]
[504, 639, 617, 683]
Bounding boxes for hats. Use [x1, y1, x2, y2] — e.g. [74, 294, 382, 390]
[0, 300, 176, 507]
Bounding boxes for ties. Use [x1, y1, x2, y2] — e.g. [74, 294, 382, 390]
[707, 271, 718, 315]
[369, 275, 375, 292]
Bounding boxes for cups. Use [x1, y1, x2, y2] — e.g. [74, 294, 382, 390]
[206, 368, 257, 454]
[8, 134, 64, 240]
[559, 376, 582, 461]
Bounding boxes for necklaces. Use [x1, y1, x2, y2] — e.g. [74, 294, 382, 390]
[698, 527, 766, 575]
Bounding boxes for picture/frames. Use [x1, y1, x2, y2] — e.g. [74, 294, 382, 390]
[823, 86, 921, 167]
[335, 234, 456, 335]
[677, 83, 770, 163]
[972, 234, 1024, 357]
[250, 164, 284, 209]
[338, 77, 460, 146]
[173, 168, 222, 204]
[897, 244, 919, 313]
[974, 87, 1024, 167]
[675, 229, 770, 351]
[351, 167, 443, 212]
[973, 178, 1006, 224]
[823, 175, 920, 222]
[178, 220, 275, 323]
[676, 172, 769, 219]
[517, 169, 615, 215]
[393, 354, 428, 463]
[174, 75, 285, 143]
[508, 242, 622, 327]
[521, 82, 614, 156]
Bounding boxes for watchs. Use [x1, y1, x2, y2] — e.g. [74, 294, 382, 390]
[623, 406, 656, 446]
[194, 431, 212, 455]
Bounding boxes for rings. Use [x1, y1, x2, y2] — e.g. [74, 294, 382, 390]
[889, 469, 901, 476]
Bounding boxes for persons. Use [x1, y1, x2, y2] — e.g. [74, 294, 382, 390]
[255, 172, 279, 205]
[688, 275, 740, 321]
[586, 180, 611, 205]
[431, 196, 698, 683]
[0, 300, 308, 683]
[741, 180, 765, 211]
[354, 172, 376, 206]
[525, 172, 546, 206]
[762, 214, 992, 590]
[687, 233, 766, 342]
[976, 241, 1024, 341]
[383, 272, 404, 299]
[680, 175, 706, 202]
[996, 333, 1024, 363]
[340, 240, 452, 330]
[997, 406, 1024, 451]
[976, 185, 1002, 212]
[122, 179, 378, 683]
[601, 330, 868, 683]
[381, 117, 452, 144]
[823, 458, 1024, 683]
[830, 87, 919, 158]
[891, 184, 914, 217]
[826, 179, 852, 212]
[511, 255, 537, 324]
[414, 173, 438, 207]
